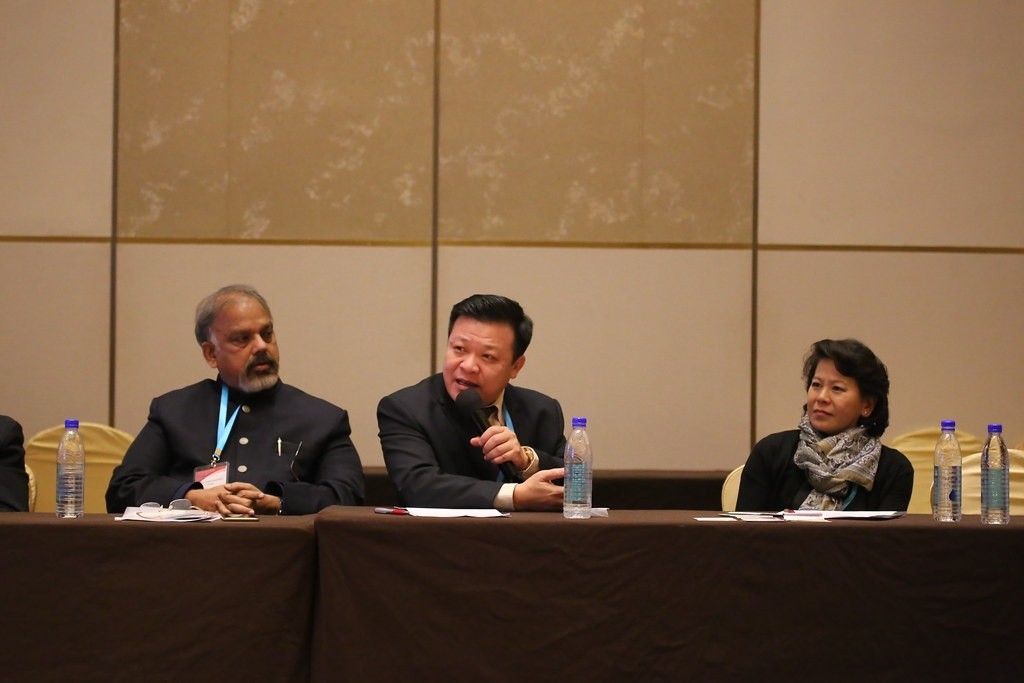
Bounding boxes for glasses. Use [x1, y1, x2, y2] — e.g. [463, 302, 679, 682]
[136, 499, 204, 518]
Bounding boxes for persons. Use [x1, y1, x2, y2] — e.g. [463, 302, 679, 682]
[376, 294, 605, 513]
[735, 337, 914, 515]
[104, 284, 368, 518]
[0, 415, 29, 512]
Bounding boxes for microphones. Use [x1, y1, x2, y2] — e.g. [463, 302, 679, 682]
[454, 389, 517, 480]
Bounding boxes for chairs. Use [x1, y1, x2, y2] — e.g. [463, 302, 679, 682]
[927, 447, 1024, 515]
[23, 422, 134, 514]
[889, 426, 986, 515]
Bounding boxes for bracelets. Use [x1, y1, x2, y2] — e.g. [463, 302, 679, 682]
[276, 495, 283, 515]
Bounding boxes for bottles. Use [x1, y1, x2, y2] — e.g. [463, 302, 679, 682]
[981, 424, 1010, 525]
[933, 421, 962, 523]
[57, 419, 84, 519]
[564, 417, 593, 518]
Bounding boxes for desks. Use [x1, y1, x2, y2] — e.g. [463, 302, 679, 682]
[362, 466, 730, 511]
[318, 504, 1024, 683]
[0, 511, 319, 683]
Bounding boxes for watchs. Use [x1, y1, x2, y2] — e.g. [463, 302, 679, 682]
[523, 447, 533, 470]
